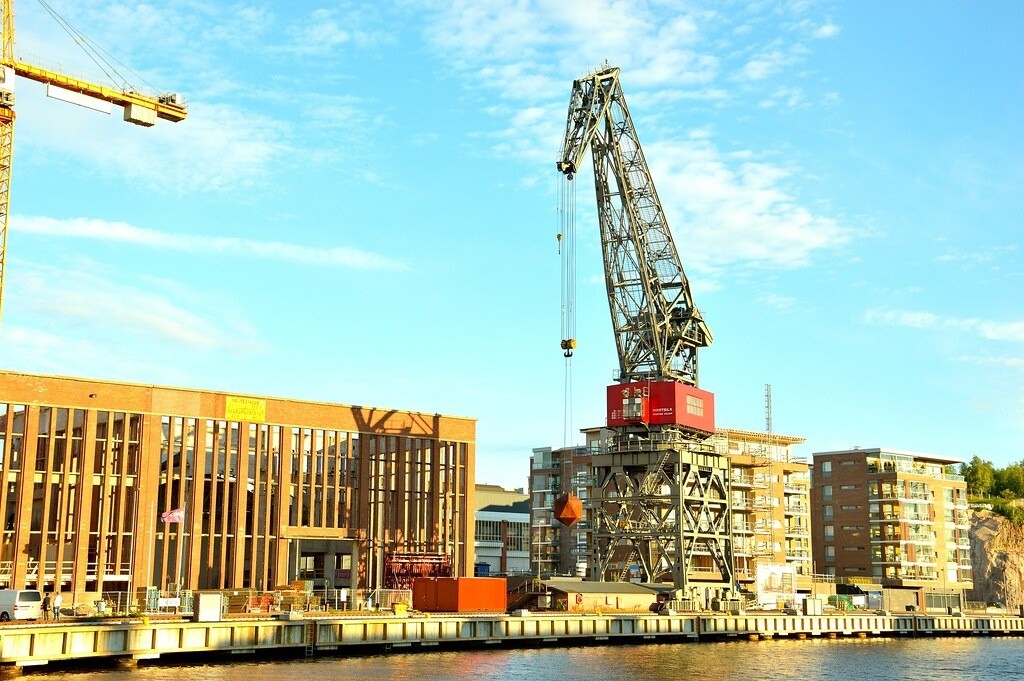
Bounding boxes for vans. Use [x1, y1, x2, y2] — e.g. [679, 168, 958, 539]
[0, 588, 45, 622]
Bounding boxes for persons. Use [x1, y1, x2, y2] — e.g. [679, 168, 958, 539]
[42, 593, 51, 620]
[53, 592, 62, 621]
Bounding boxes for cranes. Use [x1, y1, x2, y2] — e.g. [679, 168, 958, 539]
[556, 67, 741, 588]
[0, 1, 189, 306]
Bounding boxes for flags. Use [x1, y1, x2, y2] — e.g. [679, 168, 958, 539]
[161, 507, 184, 523]
